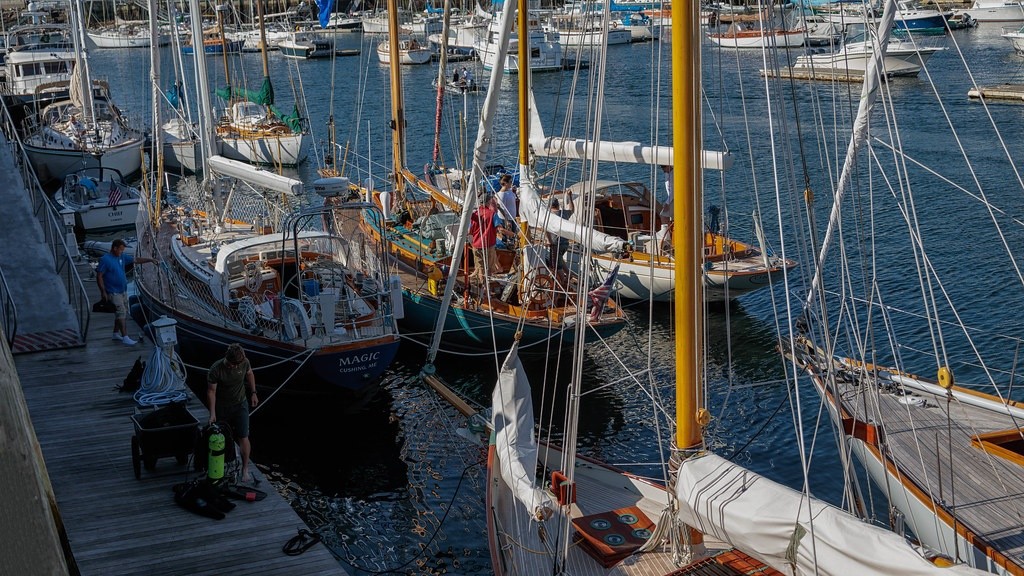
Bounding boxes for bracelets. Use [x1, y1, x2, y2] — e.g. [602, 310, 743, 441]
[251, 391, 257, 394]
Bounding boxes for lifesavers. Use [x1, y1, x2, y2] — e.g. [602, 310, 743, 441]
[280, 301, 312, 340]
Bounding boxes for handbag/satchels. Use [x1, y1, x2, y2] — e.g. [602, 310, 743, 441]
[92, 299, 117, 313]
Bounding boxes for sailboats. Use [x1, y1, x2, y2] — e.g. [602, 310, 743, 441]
[0, 1, 1024, 576]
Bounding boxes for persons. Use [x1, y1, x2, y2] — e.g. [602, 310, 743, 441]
[543, 188, 575, 284]
[58, 116, 87, 150]
[469, 173, 517, 292]
[76, 178, 100, 205]
[96, 238, 159, 345]
[208, 343, 259, 485]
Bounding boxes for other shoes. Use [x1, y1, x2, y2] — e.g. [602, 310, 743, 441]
[112, 334, 124, 341]
[123, 338, 138, 345]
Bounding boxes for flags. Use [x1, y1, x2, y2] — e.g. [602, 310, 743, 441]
[589, 264, 619, 323]
[107, 178, 122, 207]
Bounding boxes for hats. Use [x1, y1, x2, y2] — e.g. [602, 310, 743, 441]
[480, 193, 491, 203]
[549, 198, 559, 207]
[113, 239, 129, 247]
